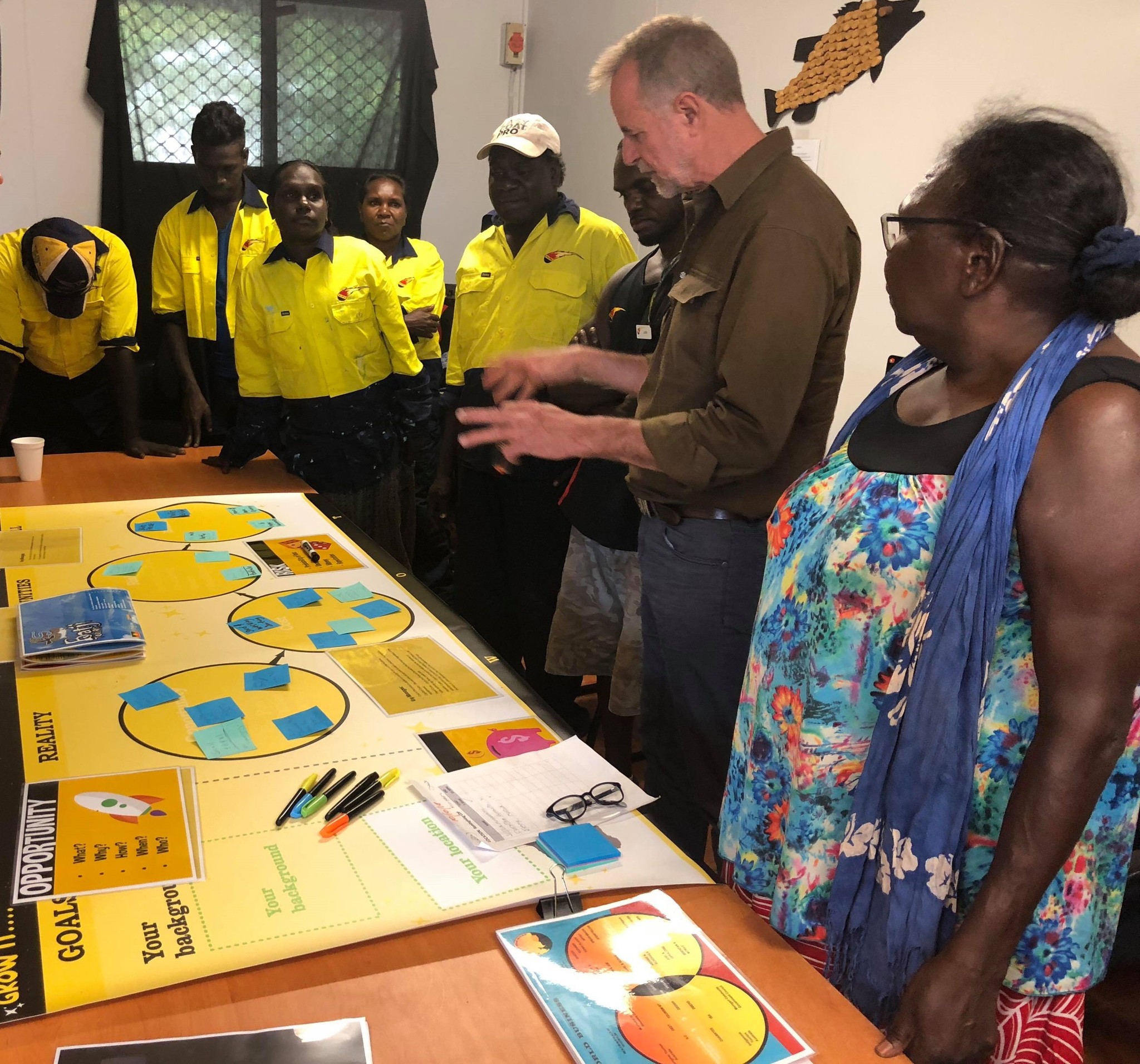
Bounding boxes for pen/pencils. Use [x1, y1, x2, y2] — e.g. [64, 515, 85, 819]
[275, 773, 318, 826]
[290, 768, 337, 818]
[341, 768, 399, 813]
[320, 790, 385, 838]
[343, 767, 398, 811]
[300, 771, 356, 818]
[325, 772, 379, 820]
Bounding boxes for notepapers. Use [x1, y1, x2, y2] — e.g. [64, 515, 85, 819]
[535, 823, 621, 874]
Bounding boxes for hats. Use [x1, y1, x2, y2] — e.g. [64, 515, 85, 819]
[474, 112, 563, 161]
[29, 217, 98, 322]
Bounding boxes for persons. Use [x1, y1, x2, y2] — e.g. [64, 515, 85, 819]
[535, 132, 696, 789]
[200, 158, 437, 578]
[151, 98, 283, 448]
[0, 218, 189, 460]
[455, 11, 864, 881]
[427, 113, 645, 700]
[347, 172, 447, 580]
[708, 101, 1138, 1063]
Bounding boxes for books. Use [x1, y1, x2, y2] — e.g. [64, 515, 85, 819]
[491, 889, 817, 1064]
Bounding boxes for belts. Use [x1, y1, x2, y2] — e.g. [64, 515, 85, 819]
[630, 487, 774, 526]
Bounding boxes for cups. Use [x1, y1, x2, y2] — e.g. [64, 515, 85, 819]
[11, 437, 45, 482]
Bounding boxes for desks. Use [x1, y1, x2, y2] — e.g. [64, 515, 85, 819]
[1, 436, 916, 1064]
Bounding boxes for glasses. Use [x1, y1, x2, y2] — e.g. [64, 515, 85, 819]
[880, 207, 1014, 256]
[543, 779, 627, 827]
